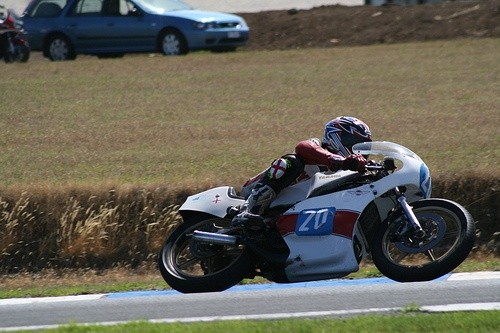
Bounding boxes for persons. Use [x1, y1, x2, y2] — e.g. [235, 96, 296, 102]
[232, 114, 373, 233]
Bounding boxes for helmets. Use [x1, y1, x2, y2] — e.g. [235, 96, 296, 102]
[324, 115, 372, 161]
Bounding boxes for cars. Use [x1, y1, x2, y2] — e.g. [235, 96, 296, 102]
[20, 0, 251, 62]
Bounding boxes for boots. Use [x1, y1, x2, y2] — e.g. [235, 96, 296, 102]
[230, 182, 276, 229]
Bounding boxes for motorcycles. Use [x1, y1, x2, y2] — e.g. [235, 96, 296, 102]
[158, 140, 476, 294]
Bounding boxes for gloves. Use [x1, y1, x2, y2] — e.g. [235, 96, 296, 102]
[329, 150, 368, 172]
